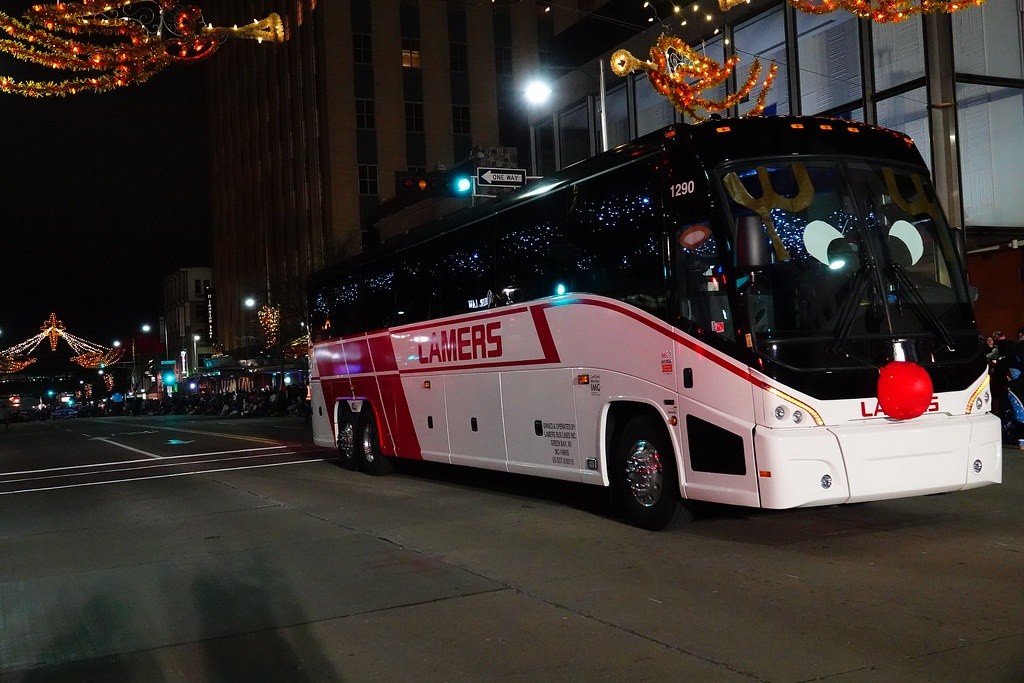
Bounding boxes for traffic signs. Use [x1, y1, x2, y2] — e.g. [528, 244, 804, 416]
[477, 167, 527, 189]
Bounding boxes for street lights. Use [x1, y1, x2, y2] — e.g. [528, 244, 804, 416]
[192, 336, 201, 376]
[524, 82, 550, 181]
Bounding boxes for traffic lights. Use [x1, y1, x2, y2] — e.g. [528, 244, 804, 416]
[396, 169, 473, 196]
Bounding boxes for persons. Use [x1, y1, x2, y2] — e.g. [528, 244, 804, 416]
[0, 383, 311, 431]
[979, 329, 1024, 444]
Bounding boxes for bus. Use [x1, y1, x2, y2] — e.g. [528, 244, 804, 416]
[302, 113, 1004, 530]
